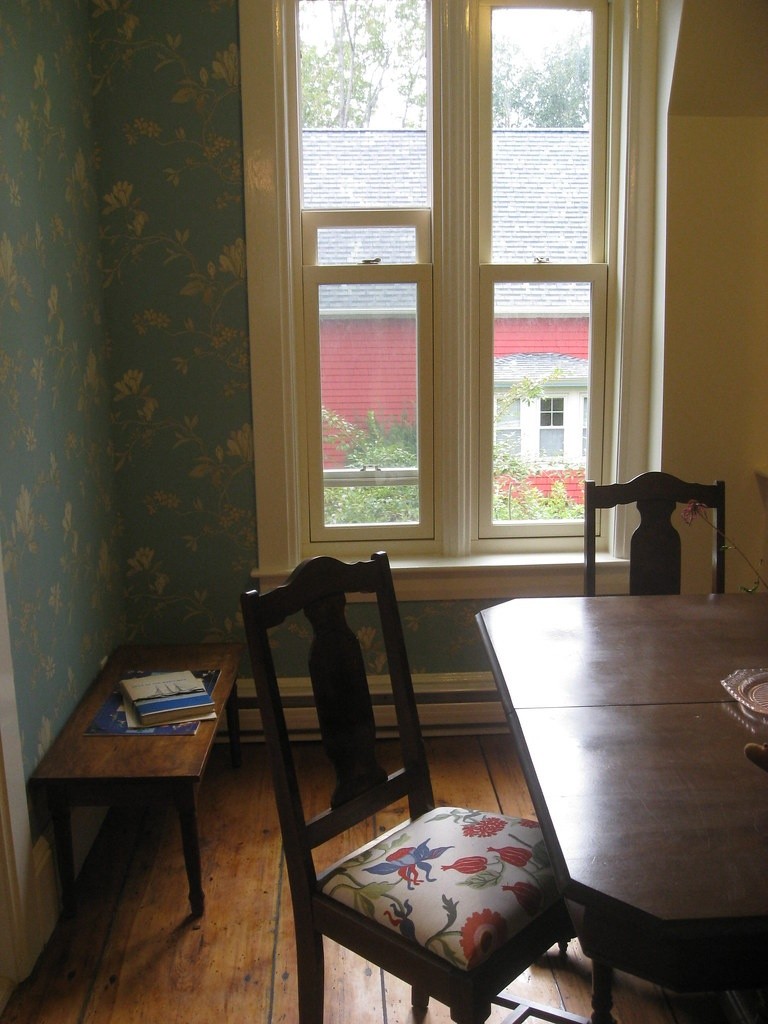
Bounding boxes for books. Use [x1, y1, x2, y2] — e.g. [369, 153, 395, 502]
[82, 664, 224, 740]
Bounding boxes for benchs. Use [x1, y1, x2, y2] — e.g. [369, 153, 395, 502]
[28, 641, 243, 917]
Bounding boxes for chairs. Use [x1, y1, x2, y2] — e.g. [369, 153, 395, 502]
[585, 471, 726, 596]
[239, 550, 579, 1024]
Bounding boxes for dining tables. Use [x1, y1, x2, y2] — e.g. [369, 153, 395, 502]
[475, 593, 768, 1024]
